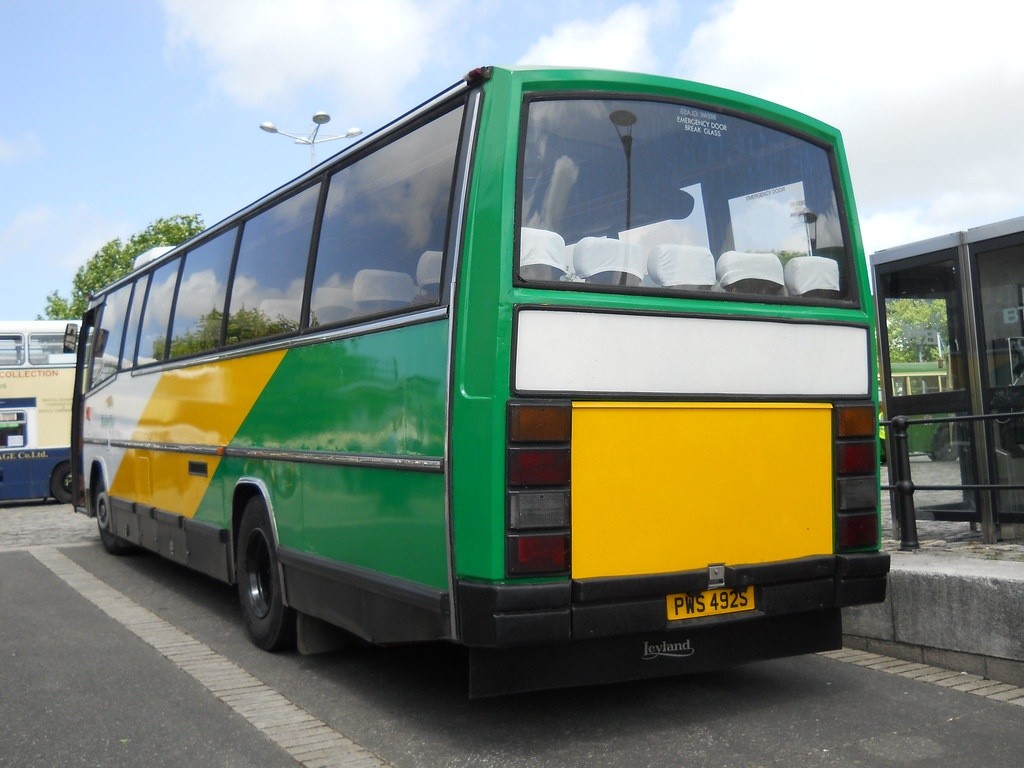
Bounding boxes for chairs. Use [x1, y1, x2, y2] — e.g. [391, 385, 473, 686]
[521, 227, 568, 281]
[350, 269, 416, 315]
[573, 237, 645, 288]
[784, 255, 843, 299]
[646, 244, 716, 290]
[256, 298, 301, 332]
[308, 287, 358, 324]
[413, 250, 443, 306]
[715, 251, 786, 296]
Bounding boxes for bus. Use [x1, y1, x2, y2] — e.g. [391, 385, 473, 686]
[0, 319, 94, 503]
[62, 65, 891, 657]
[938, 337, 1024, 459]
[875, 362, 961, 461]
[90, 353, 132, 390]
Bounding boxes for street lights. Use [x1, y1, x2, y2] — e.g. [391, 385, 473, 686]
[258, 111, 362, 167]
[799, 213, 818, 256]
[607, 112, 637, 286]
